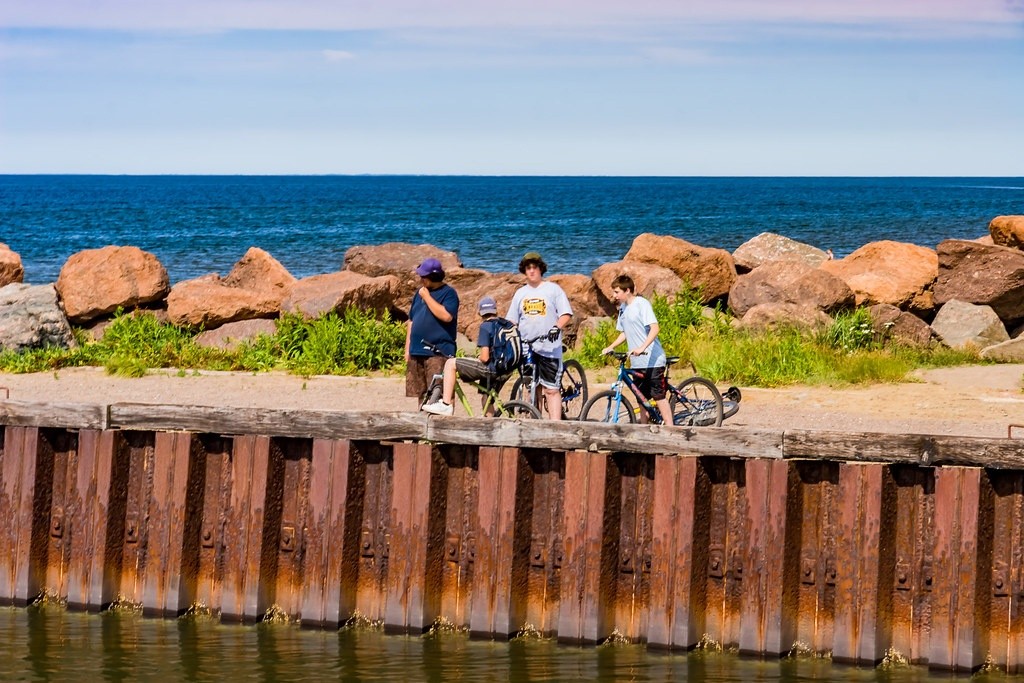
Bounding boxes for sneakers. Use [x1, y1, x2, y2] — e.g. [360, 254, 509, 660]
[422, 399, 453, 417]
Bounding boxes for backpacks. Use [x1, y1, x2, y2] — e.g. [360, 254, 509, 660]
[484, 317, 523, 373]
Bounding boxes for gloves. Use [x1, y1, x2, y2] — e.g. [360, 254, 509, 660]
[548, 325, 561, 343]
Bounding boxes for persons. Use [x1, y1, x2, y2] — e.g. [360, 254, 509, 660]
[405, 258, 460, 415]
[422, 298, 513, 416]
[602, 275, 674, 425]
[505, 253, 573, 420]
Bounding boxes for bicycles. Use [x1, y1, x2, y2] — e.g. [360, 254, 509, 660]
[418, 339, 541, 421]
[510, 333, 588, 422]
[579, 350, 724, 429]
[600, 383, 743, 430]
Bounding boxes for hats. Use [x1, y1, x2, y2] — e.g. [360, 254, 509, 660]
[518, 252, 547, 276]
[414, 258, 442, 276]
[476, 297, 497, 317]
